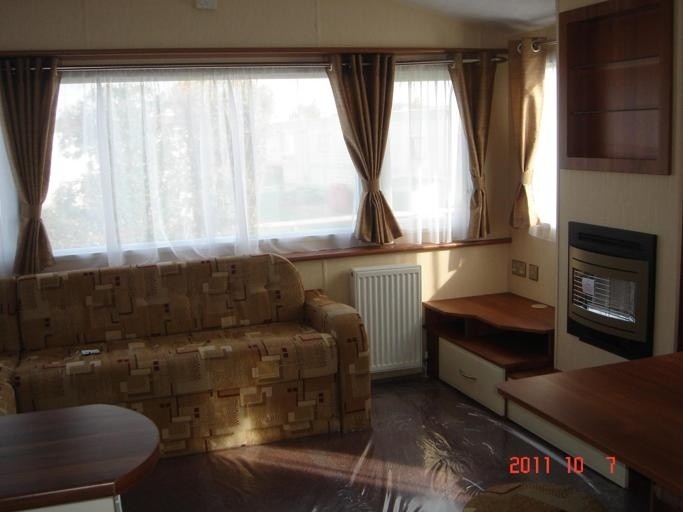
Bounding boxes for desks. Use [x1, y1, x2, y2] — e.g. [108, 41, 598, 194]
[493, 351, 682, 507]
[0, 403, 162, 509]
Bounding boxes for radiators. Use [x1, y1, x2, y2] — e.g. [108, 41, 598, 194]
[348, 264, 425, 386]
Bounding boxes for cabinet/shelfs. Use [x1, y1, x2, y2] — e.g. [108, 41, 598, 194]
[416, 286, 557, 421]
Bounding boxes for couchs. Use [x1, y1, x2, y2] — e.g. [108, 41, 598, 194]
[0, 252, 374, 462]
